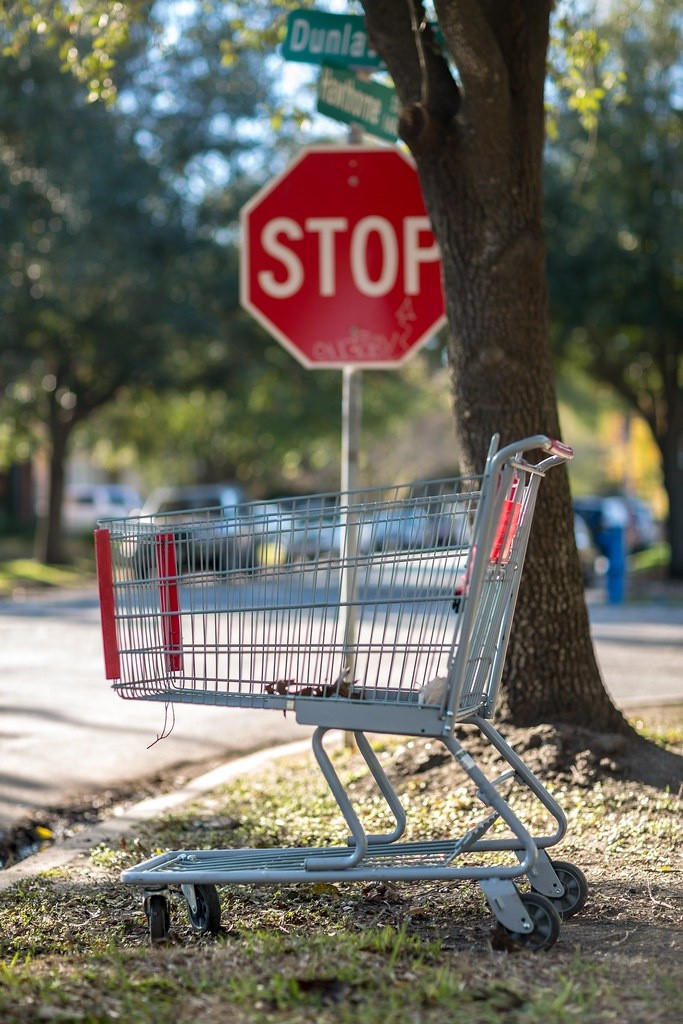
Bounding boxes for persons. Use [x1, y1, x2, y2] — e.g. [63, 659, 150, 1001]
[600, 482, 637, 605]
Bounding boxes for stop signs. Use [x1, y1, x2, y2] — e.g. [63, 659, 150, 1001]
[236, 144, 450, 373]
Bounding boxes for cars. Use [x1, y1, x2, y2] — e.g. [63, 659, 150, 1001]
[62, 481, 474, 583]
[570, 495, 654, 585]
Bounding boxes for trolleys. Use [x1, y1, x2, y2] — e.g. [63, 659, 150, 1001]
[91, 431, 589, 957]
